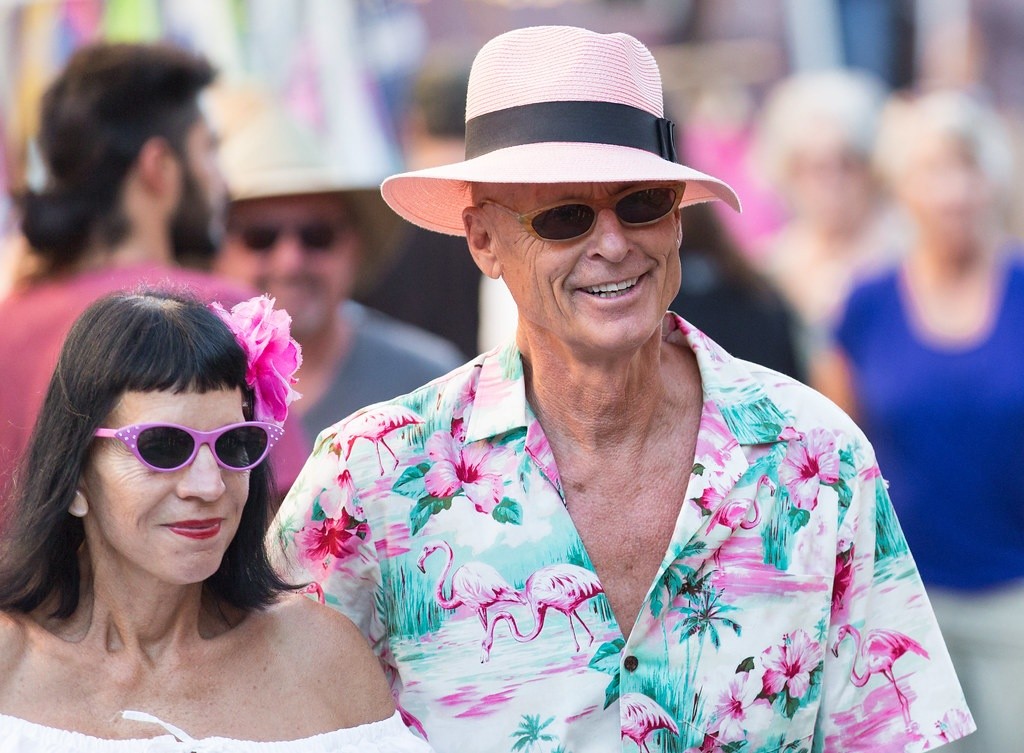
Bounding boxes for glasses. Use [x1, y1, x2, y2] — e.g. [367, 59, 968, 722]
[477, 179, 687, 243]
[234, 219, 339, 255]
[94, 421, 286, 474]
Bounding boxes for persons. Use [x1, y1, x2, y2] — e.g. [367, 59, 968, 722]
[0, 282, 434, 752]
[350, 52, 1023, 753]
[260, 26, 977, 753]
[1, 43, 462, 536]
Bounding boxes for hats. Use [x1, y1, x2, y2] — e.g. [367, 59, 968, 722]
[227, 132, 386, 204]
[379, 24, 741, 237]
